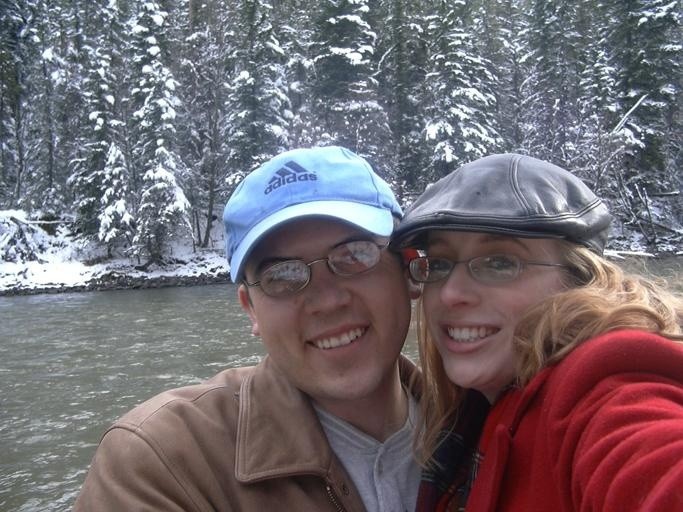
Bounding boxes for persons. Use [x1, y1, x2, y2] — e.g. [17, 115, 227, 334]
[384, 152, 683, 512]
[71, 145, 437, 511]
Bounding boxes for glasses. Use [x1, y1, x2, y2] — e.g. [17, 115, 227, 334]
[406, 255, 569, 285]
[240, 239, 392, 296]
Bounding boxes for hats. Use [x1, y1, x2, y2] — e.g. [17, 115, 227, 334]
[389, 153, 613, 258]
[222, 145, 404, 285]
